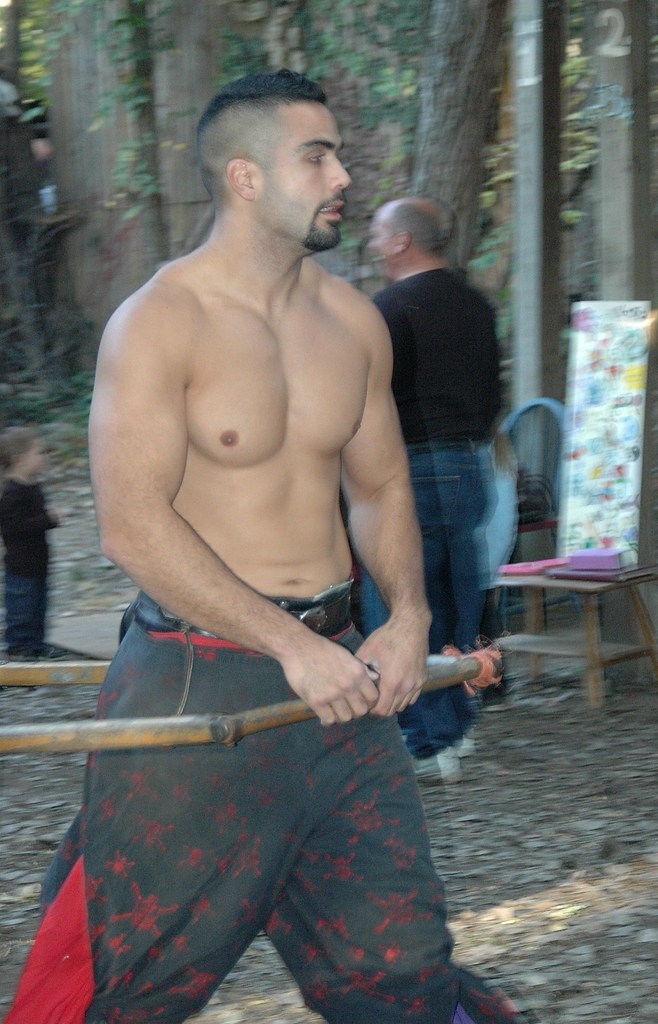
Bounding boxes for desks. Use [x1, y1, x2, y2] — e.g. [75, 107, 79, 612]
[488, 566, 658, 709]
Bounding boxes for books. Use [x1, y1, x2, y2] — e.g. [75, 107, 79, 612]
[499, 545, 658, 582]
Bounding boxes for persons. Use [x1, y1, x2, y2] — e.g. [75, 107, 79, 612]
[0, 70, 530, 1024]
[336, 197, 519, 781]
[1, 428, 69, 662]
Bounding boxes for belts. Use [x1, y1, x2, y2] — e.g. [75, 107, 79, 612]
[132, 571, 354, 638]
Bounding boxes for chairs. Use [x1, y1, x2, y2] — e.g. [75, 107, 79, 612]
[487, 399, 582, 638]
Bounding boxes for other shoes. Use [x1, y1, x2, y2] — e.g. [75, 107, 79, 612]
[401, 725, 476, 786]
